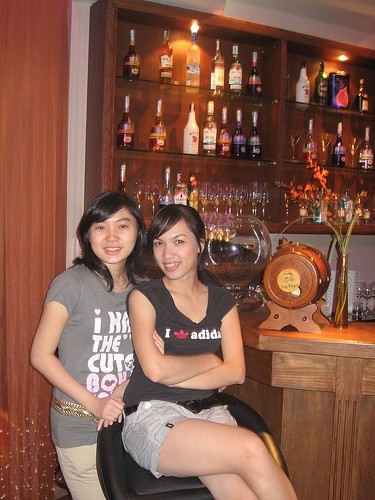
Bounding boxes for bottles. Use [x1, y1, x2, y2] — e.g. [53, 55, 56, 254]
[189, 175, 199, 211]
[116, 95, 135, 151]
[181, 103, 199, 155]
[118, 163, 127, 193]
[338, 190, 353, 224]
[202, 101, 217, 156]
[148, 100, 167, 152]
[157, 167, 175, 208]
[294, 59, 369, 114]
[227, 44, 242, 99]
[247, 111, 263, 161]
[216, 106, 232, 158]
[311, 187, 328, 224]
[122, 27, 141, 79]
[210, 39, 225, 97]
[231, 108, 246, 160]
[302, 120, 319, 165]
[246, 51, 263, 104]
[330, 122, 346, 168]
[185, 32, 200, 93]
[159, 29, 174, 83]
[358, 126, 374, 170]
[174, 172, 189, 207]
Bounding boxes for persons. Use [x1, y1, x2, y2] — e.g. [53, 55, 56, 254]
[29, 192, 150, 500]
[123, 204, 297, 500]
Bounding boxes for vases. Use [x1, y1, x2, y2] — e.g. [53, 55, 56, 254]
[331, 249, 351, 330]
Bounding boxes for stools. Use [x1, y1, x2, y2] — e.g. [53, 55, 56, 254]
[95, 392, 290, 500]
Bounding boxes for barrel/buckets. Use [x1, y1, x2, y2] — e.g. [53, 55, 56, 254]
[263, 245, 332, 309]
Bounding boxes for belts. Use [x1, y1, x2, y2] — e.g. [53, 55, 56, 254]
[51, 394, 117, 422]
[124, 393, 222, 416]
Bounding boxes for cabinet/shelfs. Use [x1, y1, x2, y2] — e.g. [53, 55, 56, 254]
[84, 0, 375, 235]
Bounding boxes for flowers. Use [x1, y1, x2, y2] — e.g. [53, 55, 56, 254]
[274, 143, 371, 325]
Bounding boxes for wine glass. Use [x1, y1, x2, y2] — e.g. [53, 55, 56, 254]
[352, 281, 375, 320]
[130, 180, 270, 228]
